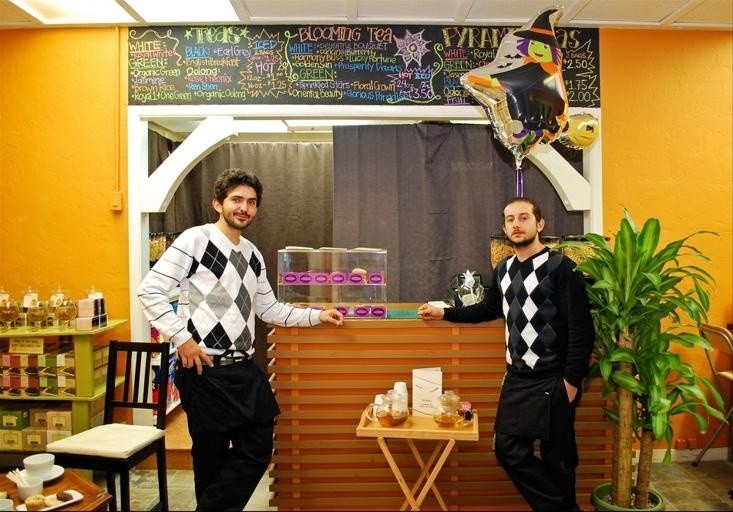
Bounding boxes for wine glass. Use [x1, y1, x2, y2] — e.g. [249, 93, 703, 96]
[0, 299, 78, 332]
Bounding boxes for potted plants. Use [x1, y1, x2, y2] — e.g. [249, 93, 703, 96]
[551, 206, 728, 512]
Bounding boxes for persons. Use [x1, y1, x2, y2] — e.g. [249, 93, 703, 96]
[134, 168, 345, 511]
[417, 198, 596, 512]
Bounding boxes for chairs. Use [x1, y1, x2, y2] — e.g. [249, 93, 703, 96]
[46, 339, 170, 512]
[691, 321, 733, 494]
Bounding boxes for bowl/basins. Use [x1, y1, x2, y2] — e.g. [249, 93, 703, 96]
[22, 452, 54, 473]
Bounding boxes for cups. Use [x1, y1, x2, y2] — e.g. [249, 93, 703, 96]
[15, 477, 44, 503]
[373, 393, 387, 419]
[0, 496, 12, 511]
[391, 380, 408, 412]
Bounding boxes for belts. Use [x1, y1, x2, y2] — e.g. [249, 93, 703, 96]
[202, 354, 252, 366]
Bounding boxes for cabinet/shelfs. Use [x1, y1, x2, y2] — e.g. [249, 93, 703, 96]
[0, 319, 129, 473]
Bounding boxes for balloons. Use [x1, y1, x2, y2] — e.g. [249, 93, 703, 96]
[458, 5, 602, 173]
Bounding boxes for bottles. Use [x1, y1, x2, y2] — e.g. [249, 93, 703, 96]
[364, 391, 409, 428]
[431, 393, 474, 429]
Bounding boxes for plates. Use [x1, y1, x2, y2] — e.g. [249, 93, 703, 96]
[15, 487, 84, 510]
[12, 463, 64, 482]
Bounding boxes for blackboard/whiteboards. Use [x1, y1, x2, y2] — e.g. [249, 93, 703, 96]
[128, 23, 602, 109]
